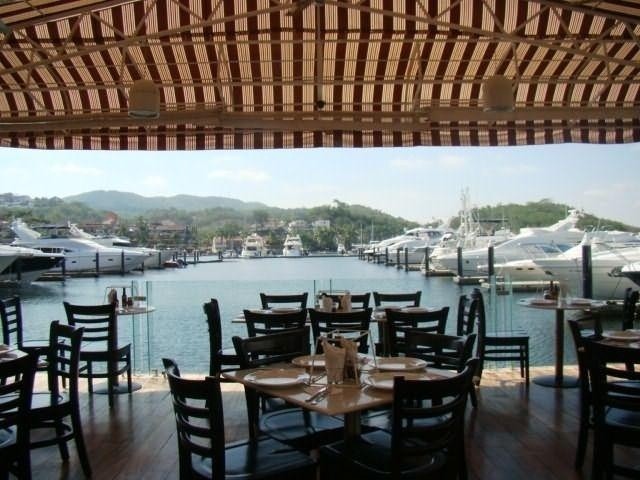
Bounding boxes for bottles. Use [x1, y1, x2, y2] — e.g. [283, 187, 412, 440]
[122, 287, 127, 307]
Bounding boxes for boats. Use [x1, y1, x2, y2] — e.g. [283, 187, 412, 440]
[64, 220, 178, 269]
[241, 233, 263, 259]
[0, 244, 66, 282]
[532, 230, 640, 301]
[8, 218, 150, 273]
[432, 208, 640, 277]
[377, 228, 443, 245]
[374, 245, 438, 264]
[477, 228, 611, 289]
[363, 239, 439, 257]
[282, 233, 303, 256]
[430, 187, 519, 270]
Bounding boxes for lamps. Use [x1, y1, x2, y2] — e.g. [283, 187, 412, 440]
[127, 78, 161, 120]
[482, 74, 515, 114]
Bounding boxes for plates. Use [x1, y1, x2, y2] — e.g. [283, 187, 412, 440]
[401, 306, 429, 313]
[528, 299, 555, 305]
[273, 306, 299, 313]
[243, 369, 309, 387]
[0, 346, 18, 355]
[291, 354, 334, 368]
[369, 356, 428, 371]
[571, 299, 595, 305]
[364, 371, 446, 391]
[602, 330, 640, 340]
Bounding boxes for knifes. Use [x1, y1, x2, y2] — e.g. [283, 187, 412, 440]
[305, 386, 328, 402]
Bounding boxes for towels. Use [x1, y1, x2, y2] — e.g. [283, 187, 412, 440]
[321, 337, 346, 382]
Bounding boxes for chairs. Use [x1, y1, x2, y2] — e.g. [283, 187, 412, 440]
[373, 290, 422, 357]
[622, 287, 639, 379]
[0, 348, 41, 480]
[62, 301, 133, 407]
[454, 294, 479, 409]
[580, 336, 640, 480]
[470, 288, 530, 386]
[203, 297, 251, 409]
[321, 291, 370, 348]
[361, 321, 478, 431]
[0, 293, 67, 392]
[384, 305, 451, 364]
[161, 357, 317, 480]
[567, 310, 640, 471]
[242, 307, 308, 360]
[231, 325, 345, 443]
[321, 356, 481, 480]
[0, 319, 94, 480]
[259, 292, 308, 329]
[308, 306, 373, 355]
